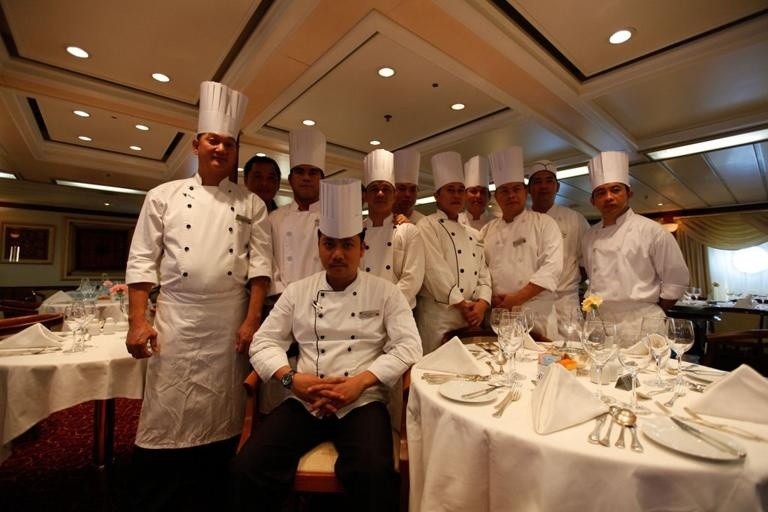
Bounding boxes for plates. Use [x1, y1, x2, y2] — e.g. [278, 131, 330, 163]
[440, 381, 497, 402]
[643, 418, 746, 461]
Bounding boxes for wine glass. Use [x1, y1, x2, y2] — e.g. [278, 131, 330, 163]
[684, 287, 694, 304]
[64, 279, 99, 354]
[490, 308, 695, 402]
[694, 288, 701, 302]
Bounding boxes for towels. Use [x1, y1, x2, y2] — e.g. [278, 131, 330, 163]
[744, 294, 753, 308]
[416, 337, 492, 378]
[622, 334, 671, 368]
[689, 363, 767, 425]
[522, 301, 558, 350]
[42, 291, 73, 304]
[0, 322, 65, 349]
[531, 363, 608, 436]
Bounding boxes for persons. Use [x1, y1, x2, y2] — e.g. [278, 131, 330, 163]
[414, 149, 493, 355]
[478, 145, 565, 341]
[526, 157, 591, 321]
[457, 154, 499, 231]
[243, 154, 282, 215]
[125, 80, 273, 512]
[358, 149, 426, 311]
[390, 147, 427, 227]
[258, 125, 327, 305]
[240, 174, 426, 512]
[580, 150, 691, 358]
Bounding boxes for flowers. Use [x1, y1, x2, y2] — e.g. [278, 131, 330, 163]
[110, 285, 128, 313]
[581, 293, 625, 367]
[102, 280, 113, 294]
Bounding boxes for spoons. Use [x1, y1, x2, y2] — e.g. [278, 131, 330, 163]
[600, 406, 622, 446]
[615, 409, 636, 450]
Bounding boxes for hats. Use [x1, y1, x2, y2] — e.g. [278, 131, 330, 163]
[363, 149, 396, 190]
[529, 160, 556, 182]
[195, 81, 248, 143]
[588, 151, 630, 194]
[488, 147, 524, 189]
[289, 128, 326, 178]
[318, 178, 364, 239]
[394, 150, 421, 186]
[464, 156, 489, 191]
[431, 151, 465, 196]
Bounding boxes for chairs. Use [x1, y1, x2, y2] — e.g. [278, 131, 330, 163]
[236, 339, 405, 492]
[705, 306, 768, 372]
[0, 296, 63, 336]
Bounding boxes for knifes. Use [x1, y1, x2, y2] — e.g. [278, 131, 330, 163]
[671, 415, 746, 458]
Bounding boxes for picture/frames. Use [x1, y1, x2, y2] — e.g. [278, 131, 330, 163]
[0, 221, 57, 265]
[60, 215, 137, 281]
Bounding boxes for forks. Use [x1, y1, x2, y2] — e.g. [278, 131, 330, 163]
[0, 348, 45, 354]
[492, 382, 521, 418]
[685, 406, 763, 443]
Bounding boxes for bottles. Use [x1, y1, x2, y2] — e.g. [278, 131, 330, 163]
[92, 317, 115, 335]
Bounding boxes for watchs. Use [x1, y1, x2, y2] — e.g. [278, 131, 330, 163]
[281, 369, 297, 390]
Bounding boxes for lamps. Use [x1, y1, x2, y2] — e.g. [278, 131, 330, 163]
[660, 215, 678, 233]
